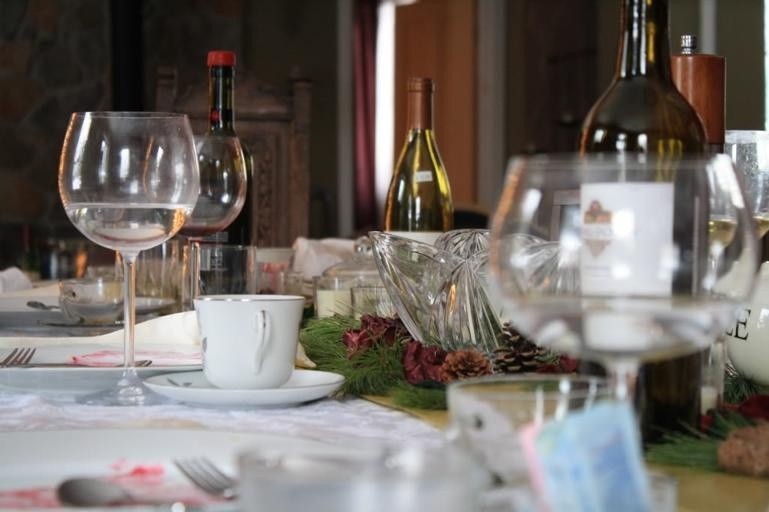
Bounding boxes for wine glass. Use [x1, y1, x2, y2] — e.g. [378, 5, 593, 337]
[56, 108, 203, 407]
[480, 152, 760, 512]
[140, 136, 252, 310]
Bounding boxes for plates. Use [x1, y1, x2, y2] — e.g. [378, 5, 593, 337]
[141, 368, 348, 410]
[0, 337, 203, 396]
[0, 294, 176, 328]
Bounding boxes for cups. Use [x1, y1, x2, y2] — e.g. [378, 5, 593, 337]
[444, 369, 627, 512]
[58, 278, 126, 336]
[192, 294, 305, 388]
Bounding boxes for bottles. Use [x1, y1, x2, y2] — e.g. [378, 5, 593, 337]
[579, 0, 711, 455]
[384, 72, 454, 232]
[187, 48, 254, 294]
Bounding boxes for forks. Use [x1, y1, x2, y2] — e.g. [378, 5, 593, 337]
[0, 348, 152, 368]
